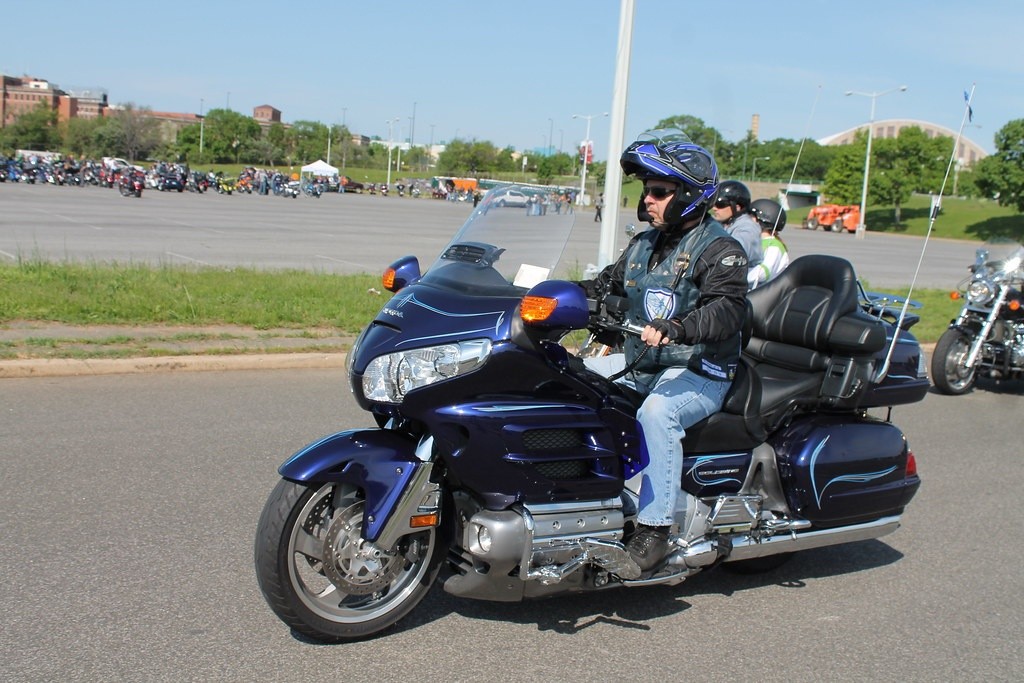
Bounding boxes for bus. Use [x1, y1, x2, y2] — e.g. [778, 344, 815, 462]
[430, 176, 477, 191]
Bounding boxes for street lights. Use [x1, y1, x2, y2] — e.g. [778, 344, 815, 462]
[752, 157, 770, 182]
[573, 113, 608, 205]
[844, 85, 908, 241]
[386, 117, 400, 190]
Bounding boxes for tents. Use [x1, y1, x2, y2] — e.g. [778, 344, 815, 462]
[300, 159, 338, 183]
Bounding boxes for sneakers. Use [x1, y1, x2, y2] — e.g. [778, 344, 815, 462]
[625, 525, 670, 571]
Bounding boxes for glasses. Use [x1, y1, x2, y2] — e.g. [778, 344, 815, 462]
[642, 185, 677, 199]
[715, 199, 741, 208]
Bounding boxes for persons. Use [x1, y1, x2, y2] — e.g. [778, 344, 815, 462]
[746, 199, 790, 290]
[593, 192, 604, 221]
[565, 127, 751, 580]
[526, 192, 575, 216]
[258, 170, 268, 194]
[337, 176, 348, 193]
[710, 180, 763, 268]
[446, 184, 479, 207]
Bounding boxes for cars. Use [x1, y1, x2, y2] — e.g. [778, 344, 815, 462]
[330, 177, 363, 192]
[492, 190, 531, 208]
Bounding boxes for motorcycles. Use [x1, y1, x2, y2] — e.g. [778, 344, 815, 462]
[254, 182, 931, 639]
[932, 237, 1024, 396]
[397, 185, 405, 196]
[302, 178, 323, 197]
[279, 177, 300, 199]
[380, 184, 389, 196]
[118, 167, 145, 197]
[1, 152, 254, 195]
[368, 183, 376, 195]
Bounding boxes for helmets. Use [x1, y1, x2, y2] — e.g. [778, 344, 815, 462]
[620, 140, 719, 227]
[718, 180, 750, 210]
[750, 199, 786, 231]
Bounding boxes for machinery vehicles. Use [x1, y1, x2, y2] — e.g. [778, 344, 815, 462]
[802, 203, 861, 234]
